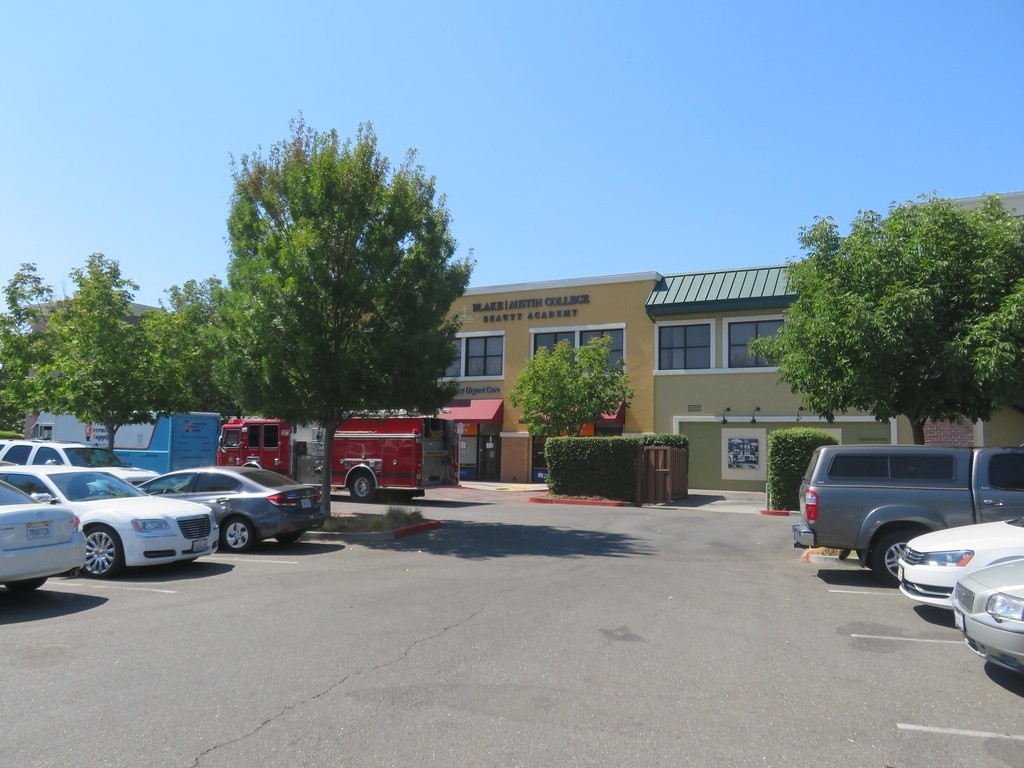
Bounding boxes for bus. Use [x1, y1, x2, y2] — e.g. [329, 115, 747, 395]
[30, 409, 222, 476]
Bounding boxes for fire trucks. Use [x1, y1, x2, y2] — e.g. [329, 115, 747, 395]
[216, 415, 462, 504]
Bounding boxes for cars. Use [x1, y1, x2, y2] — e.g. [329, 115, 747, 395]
[135, 466, 326, 554]
[896, 516, 1024, 610]
[951, 559, 1024, 673]
[0, 480, 88, 592]
[791, 445, 1024, 587]
[0, 463, 219, 579]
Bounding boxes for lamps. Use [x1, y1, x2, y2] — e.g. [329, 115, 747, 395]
[721, 407, 730, 424]
[750, 407, 760, 423]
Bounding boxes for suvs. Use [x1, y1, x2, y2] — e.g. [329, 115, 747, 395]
[0, 438, 160, 486]
